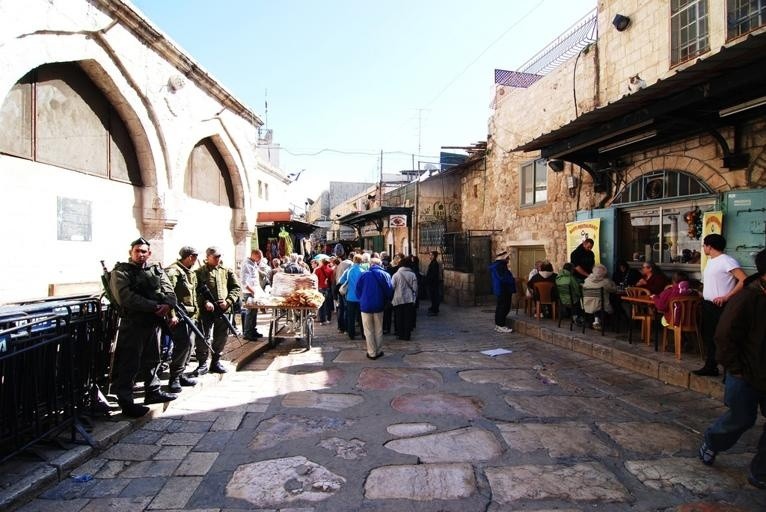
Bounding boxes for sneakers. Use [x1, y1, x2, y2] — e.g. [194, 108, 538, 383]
[698, 442, 716, 464]
[320, 320, 411, 360]
[245, 334, 257, 341]
[748, 473, 765, 488]
[493, 312, 544, 333]
[252, 331, 262, 337]
[573, 316, 601, 330]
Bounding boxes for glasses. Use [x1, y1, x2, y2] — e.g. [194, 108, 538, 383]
[642, 266, 650, 269]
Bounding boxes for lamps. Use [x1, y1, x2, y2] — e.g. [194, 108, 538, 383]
[610, 13, 629, 32]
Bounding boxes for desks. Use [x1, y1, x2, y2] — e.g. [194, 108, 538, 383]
[622, 293, 704, 353]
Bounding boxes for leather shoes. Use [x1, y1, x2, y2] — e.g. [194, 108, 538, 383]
[209, 362, 227, 373]
[180, 373, 197, 386]
[188, 362, 208, 378]
[168, 377, 182, 392]
[691, 367, 720, 377]
[122, 404, 149, 417]
[144, 390, 178, 405]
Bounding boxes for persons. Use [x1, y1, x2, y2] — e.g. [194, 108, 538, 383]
[159, 246, 198, 392]
[489, 248, 517, 332]
[427, 250, 440, 311]
[259, 252, 310, 317]
[527, 239, 691, 351]
[693, 234, 747, 376]
[193, 246, 239, 376]
[699, 249, 766, 490]
[110, 237, 177, 417]
[312, 242, 420, 342]
[241, 249, 262, 340]
[355, 259, 393, 359]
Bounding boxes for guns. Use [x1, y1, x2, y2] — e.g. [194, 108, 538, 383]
[175, 304, 217, 357]
[201, 279, 244, 344]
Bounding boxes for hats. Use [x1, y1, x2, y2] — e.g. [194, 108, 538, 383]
[206, 246, 221, 257]
[179, 246, 198, 259]
[132, 237, 149, 245]
[494, 248, 511, 261]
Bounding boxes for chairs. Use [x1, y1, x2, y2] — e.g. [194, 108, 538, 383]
[622, 286, 668, 351]
[662, 294, 706, 364]
[554, 282, 583, 333]
[513, 275, 537, 316]
[531, 278, 562, 323]
[579, 285, 620, 337]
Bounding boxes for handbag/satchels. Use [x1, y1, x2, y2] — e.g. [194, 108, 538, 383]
[338, 266, 355, 295]
